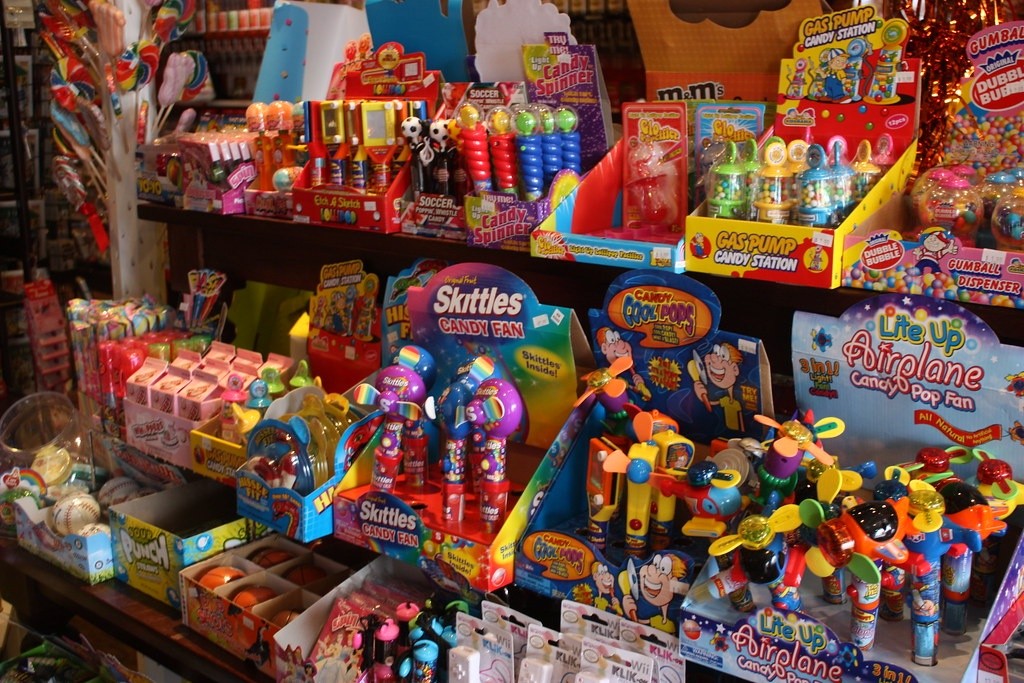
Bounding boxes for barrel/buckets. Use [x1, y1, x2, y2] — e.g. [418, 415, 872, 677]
[0, 392, 93, 540]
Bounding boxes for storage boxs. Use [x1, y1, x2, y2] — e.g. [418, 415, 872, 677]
[108, 478, 383, 679]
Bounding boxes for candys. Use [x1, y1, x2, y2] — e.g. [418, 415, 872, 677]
[38, 0, 209, 210]
[707, 112, 1024, 241]
[842, 262, 1024, 309]
